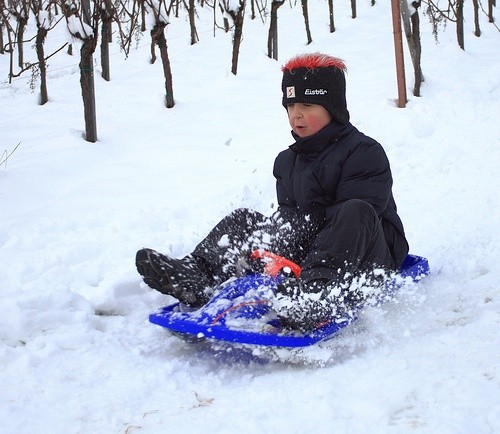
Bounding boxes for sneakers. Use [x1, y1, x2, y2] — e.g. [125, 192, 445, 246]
[135, 248, 211, 306]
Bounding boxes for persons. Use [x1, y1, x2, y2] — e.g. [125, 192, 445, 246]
[135, 54, 409, 330]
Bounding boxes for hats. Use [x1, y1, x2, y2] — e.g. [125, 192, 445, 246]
[281, 52, 351, 124]
